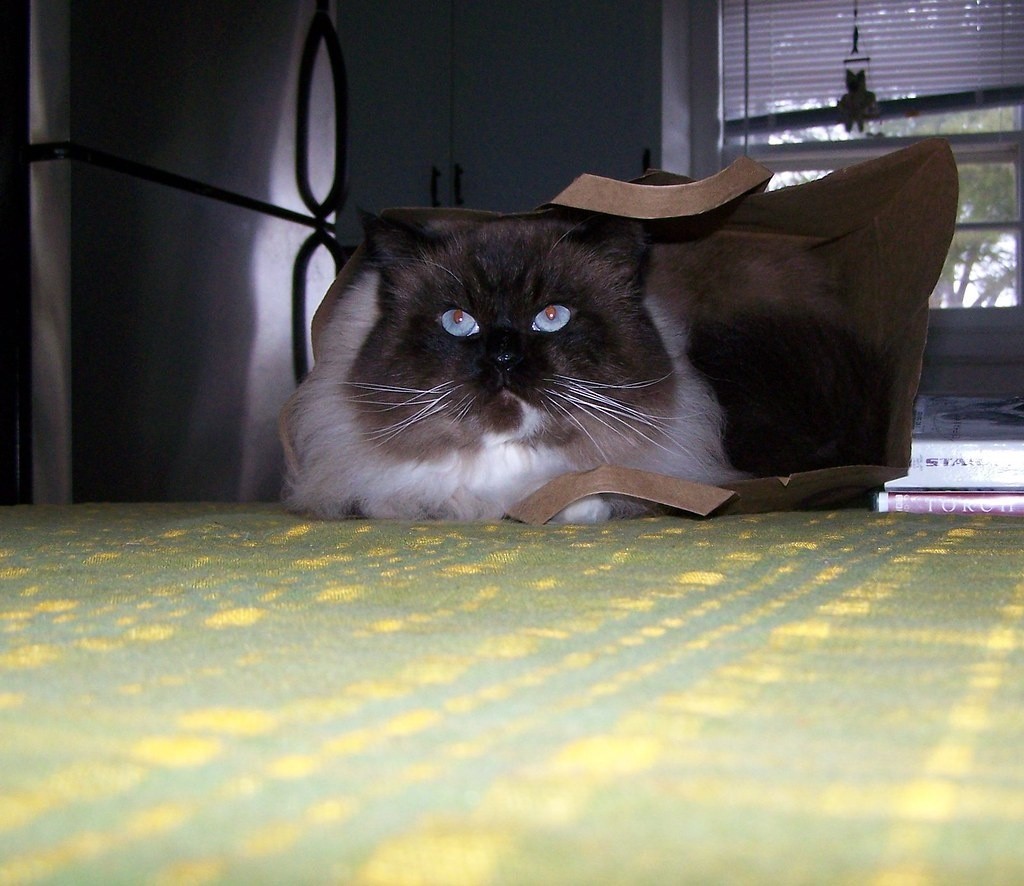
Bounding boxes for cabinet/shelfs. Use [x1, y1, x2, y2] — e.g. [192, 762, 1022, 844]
[331, 1, 692, 245]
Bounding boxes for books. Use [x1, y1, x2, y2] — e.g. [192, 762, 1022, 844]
[879, 394, 1024, 516]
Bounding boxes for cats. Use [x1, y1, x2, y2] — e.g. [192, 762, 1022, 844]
[285, 203, 733, 525]
